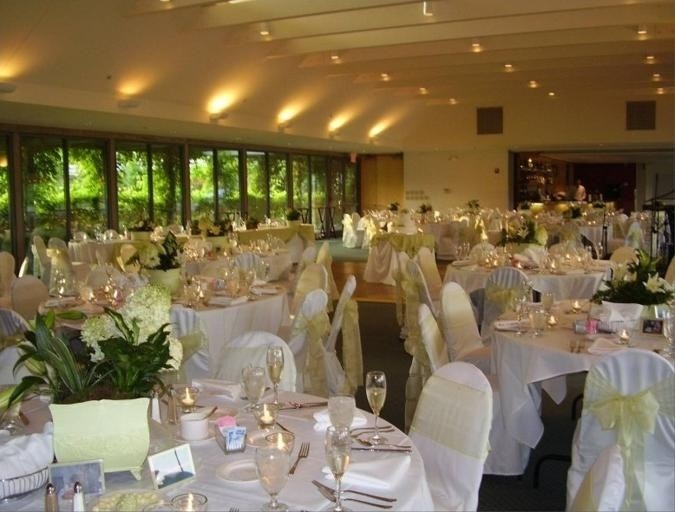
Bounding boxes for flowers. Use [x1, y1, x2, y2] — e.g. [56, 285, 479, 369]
[387, 200, 400, 210]
[592, 200, 607, 209]
[467, 199, 481, 211]
[416, 203, 431, 212]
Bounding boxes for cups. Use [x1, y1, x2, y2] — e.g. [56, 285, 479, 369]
[0, 383, 22, 434]
[176, 385, 200, 413]
[143, 500, 173, 511]
[254, 401, 279, 430]
[179, 412, 209, 439]
[170, 492, 208, 511]
[264, 431, 296, 453]
[328, 395, 356, 429]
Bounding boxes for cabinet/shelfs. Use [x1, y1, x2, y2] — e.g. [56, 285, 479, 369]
[520, 171, 553, 201]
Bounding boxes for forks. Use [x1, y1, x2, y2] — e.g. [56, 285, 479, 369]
[228, 503, 241, 511]
[312, 479, 397, 501]
[287, 443, 312, 476]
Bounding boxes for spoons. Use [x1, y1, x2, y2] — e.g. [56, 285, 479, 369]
[319, 486, 393, 508]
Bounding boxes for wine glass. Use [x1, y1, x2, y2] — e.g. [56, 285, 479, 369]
[323, 424, 353, 511]
[364, 371, 389, 443]
[266, 342, 284, 408]
[241, 366, 266, 412]
[256, 446, 289, 512]
[36, 196, 674, 355]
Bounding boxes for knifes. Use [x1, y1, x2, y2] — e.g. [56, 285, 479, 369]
[350, 446, 412, 454]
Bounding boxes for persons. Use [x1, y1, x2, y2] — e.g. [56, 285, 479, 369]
[568, 179, 587, 201]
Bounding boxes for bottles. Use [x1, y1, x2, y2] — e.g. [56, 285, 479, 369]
[45, 483, 59, 511]
[73, 479, 84, 511]
[151, 390, 161, 424]
[167, 388, 179, 423]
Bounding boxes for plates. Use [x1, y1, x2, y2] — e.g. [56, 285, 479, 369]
[196, 405, 241, 424]
[84, 488, 171, 511]
[246, 430, 270, 446]
[216, 457, 261, 483]
[168, 428, 215, 443]
